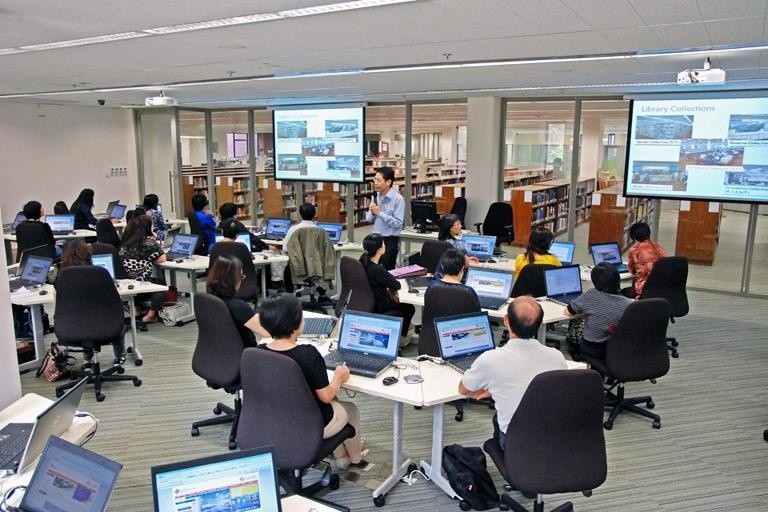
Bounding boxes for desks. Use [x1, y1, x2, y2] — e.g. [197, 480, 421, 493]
[7, 263, 168, 373]
[396, 227, 477, 266]
[245, 224, 369, 305]
[466, 254, 634, 281]
[95, 213, 184, 232]
[256, 310, 591, 511]
[378, 272, 585, 347]
[0, 393, 97, 511]
[153, 246, 290, 327]
[2, 225, 98, 263]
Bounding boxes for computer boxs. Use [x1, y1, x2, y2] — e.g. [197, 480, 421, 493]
[403, 251, 420, 266]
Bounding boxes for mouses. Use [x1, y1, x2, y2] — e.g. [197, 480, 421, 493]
[176, 258, 184, 263]
[39, 291, 48, 295]
[276, 238, 283, 241]
[383, 377, 398, 385]
[264, 255, 268, 259]
[338, 244, 343, 246]
[128, 285, 134, 289]
[488, 259, 496, 263]
[408, 288, 419, 293]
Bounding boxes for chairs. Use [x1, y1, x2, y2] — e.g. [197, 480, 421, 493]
[92, 243, 147, 333]
[191, 292, 266, 450]
[53, 201, 70, 214]
[50, 266, 142, 405]
[578, 298, 669, 432]
[332, 254, 399, 316]
[636, 256, 689, 359]
[483, 369, 610, 512]
[93, 217, 122, 248]
[418, 241, 457, 272]
[474, 201, 514, 245]
[410, 283, 495, 421]
[447, 197, 466, 230]
[236, 346, 355, 512]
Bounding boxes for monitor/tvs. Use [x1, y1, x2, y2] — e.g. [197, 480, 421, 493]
[411, 201, 437, 233]
[11, 212, 28, 230]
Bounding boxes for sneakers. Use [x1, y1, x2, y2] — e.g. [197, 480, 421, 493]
[114, 357, 126, 363]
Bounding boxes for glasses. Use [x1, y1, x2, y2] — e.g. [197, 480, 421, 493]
[240, 274, 245, 279]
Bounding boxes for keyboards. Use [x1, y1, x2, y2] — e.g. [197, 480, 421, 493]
[414, 227, 437, 231]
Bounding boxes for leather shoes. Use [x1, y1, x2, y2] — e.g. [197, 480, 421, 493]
[143, 317, 158, 323]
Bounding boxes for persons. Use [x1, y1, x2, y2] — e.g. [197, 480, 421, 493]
[437, 213, 480, 264]
[218, 202, 279, 252]
[563, 261, 635, 360]
[54, 237, 128, 365]
[204, 253, 272, 347]
[513, 226, 564, 287]
[119, 206, 158, 244]
[68, 188, 123, 233]
[191, 193, 220, 254]
[458, 295, 570, 452]
[365, 166, 406, 272]
[16, 201, 63, 265]
[118, 214, 166, 324]
[545, 157, 567, 180]
[424, 247, 482, 311]
[255, 291, 370, 470]
[208, 219, 256, 277]
[259, 149, 268, 160]
[618, 222, 668, 300]
[142, 194, 178, 243]
[359, 232, 416, 349]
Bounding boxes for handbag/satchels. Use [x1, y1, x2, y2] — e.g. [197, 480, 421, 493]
[158, 302, 191, 327]
[442, 443, 500, 511]
[37, 341, 77, 382]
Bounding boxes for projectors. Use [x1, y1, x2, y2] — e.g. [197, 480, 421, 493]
[677, 68, 726, 86]
[145, 96, 177, 107]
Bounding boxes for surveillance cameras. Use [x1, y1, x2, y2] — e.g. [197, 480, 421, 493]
[98, 100, 105, 105]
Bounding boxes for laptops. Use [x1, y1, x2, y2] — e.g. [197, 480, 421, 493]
[91, 254, 119, 287]
[257, 218, 292, 240]
[432, 311, 496, 374]
[316, 223, 344, 245]
[45, 213, 75, 235]
[464, 266, 515, 310]
[406, 255, 444, 289]
[590, 242, 629, 273]
[214, 233, 255, 260]
[542, 264, 582, 306]
[547, 242, 576, 265]
[294, 289, 353, 338]
[96, 200, 120, 216]
[136, 204, 162, 213]
[0, 375, 89, 474]
[462, 234, 498, 262]
[9, 255, 54, 293]
[151, 444, 282, 512]
[160, 227, 181, 249]
[0, 434, 124, 512]
[165, 233, 200, 261]
[323, 309, 404, 378]
[109, 204, 127, 221]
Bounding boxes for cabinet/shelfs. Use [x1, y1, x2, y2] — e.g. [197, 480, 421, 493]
[263, 164, 273, 176]
[316, 177, 377, 230]
[503, 167, 553, 189]
[508, 176, 597, 245]
[588, 183, 656, 254]
[181, 164, 262, 222]
[364, 153, 463, 176]
[393, 174, 464, 214]
[673, 200, 723, 267]
[433, 181, 464, 214]
[264, 178, 314, 224]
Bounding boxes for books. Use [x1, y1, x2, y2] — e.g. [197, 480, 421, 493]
[353, 196, 365, 210]
[283, 209, 297, 220]
[393, 185, 405, 198]
[233, 191, 264, 204]
[365, 194, 376, 209]
[365, 181, 375, 194]
[576, 206, 592, 224]
[576, 195, 592, 208]
[533, 185, 570, 204]
[233, 176, 265, 190]
[353, 210, 365, 224]
[235, 205, 263, 216]
[281, 202, 332, 303]
[625, 198, 653, 223]
[623, 231, 633, 249]
[412, 183, 433, 198]
[340, 183, 347, 211]
[539, 218, 568, 233]
[353, 183, 364, 194]
[282, 182, 317, 207]
[577, 178, 595, 196]
[193, 177, 207, 193]
[531, 201, 569, 222]
